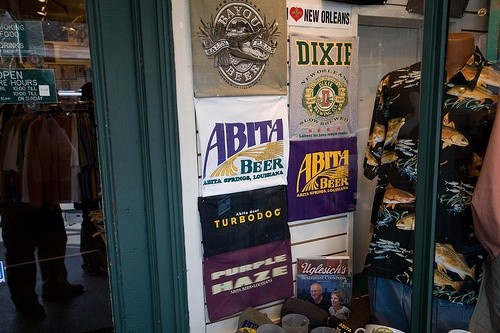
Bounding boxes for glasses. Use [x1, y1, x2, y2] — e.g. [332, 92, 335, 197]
[310, 290, 318, 293]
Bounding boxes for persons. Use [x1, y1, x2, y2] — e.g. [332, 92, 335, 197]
[0, 59, 108, 324]
[329, 290, 351, 319]
[308, 283, 333, 318]
[361, 31, 500, 332]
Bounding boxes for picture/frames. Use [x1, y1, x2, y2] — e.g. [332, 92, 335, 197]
[42, 20, 89, 91]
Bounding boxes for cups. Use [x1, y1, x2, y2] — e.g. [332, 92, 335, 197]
[355, 324, 405, 333]
[311, 326, 337, 333]
[281, 313, 309, 333]
[257, 323, 282, 333]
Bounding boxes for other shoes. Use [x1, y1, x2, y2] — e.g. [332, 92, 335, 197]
[42, 284, 84, 300]
[81, 261, 108, 273]
[15, 303, 47, 320]
